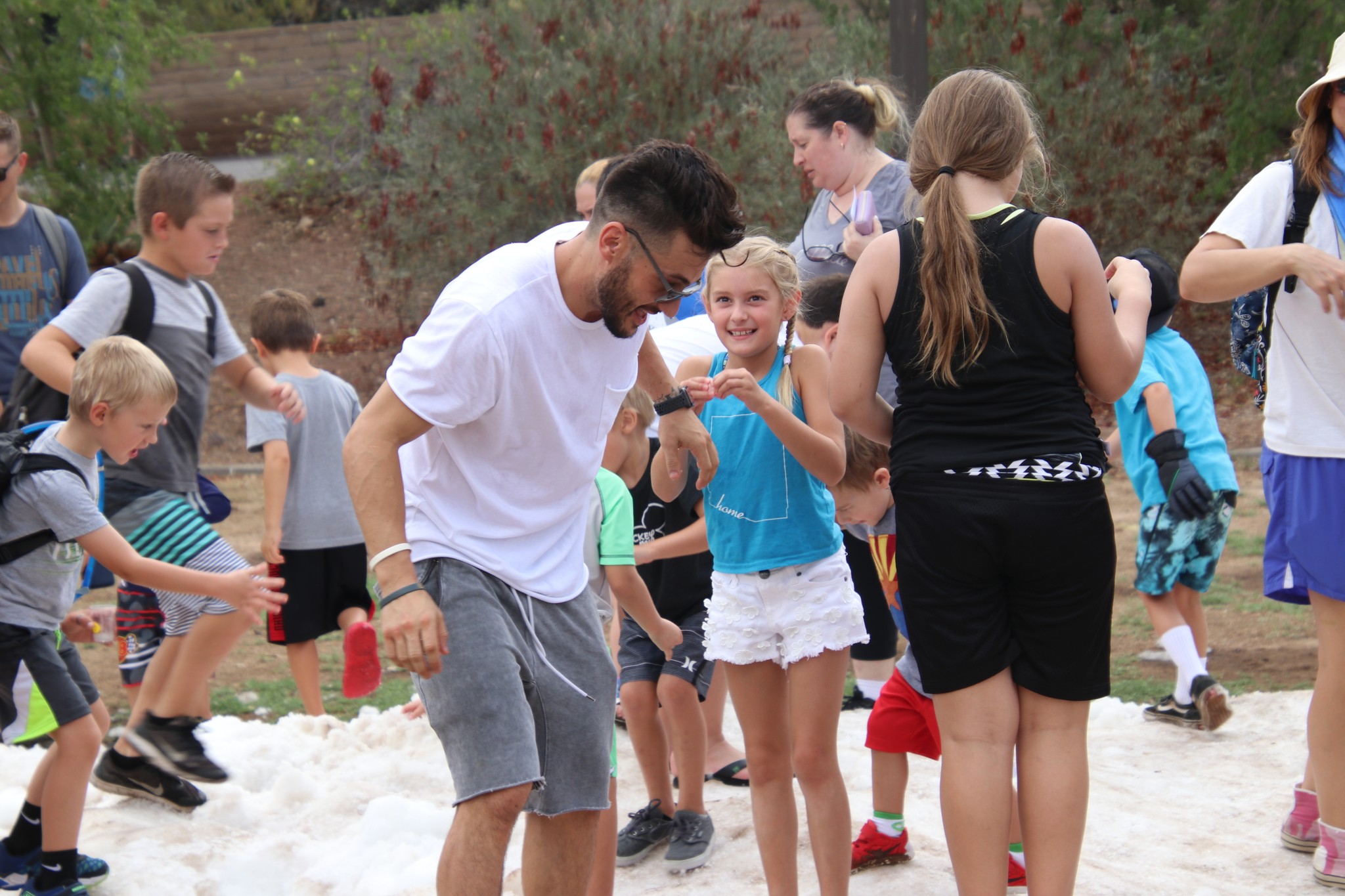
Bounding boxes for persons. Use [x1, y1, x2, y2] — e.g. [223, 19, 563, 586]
[338, 75, 1157, 896]
[1110, 251, 1240, 736]
[1179, 32, 1345, 896]
[0, 107, 306, 896]
[247, 286, 382, 718]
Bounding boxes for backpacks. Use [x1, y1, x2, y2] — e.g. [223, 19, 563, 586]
[0, 418, 91, 564]
[5, 252, 219, 412]
[1229, 135, 1321, 410]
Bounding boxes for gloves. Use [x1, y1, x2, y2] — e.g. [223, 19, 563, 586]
[1145, 429, 1213, 522]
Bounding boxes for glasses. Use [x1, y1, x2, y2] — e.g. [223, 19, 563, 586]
[0, 154, 19, 181]
[625, 226, 702, 304]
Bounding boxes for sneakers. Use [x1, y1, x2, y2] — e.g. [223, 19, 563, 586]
[124, 708, 227, 780]
[1281, 781, 1345, 889]
[615, 798, 677, 865]
[342, 621, 381, 699]
[665, 808, 718, 868]
[89, 747, 207, 812]
[0, 837, 109, 890]
[1189, 674, 1233, 731]
[1142, 694, 1202, 729]
[20, 876, 88, 896]
[1009, 852, 1028, 895]
[850, 819, 914, 873]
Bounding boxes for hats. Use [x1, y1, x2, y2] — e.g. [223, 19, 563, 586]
[1118, 246, 1180, 332]
[1297, 30, 1345, 124]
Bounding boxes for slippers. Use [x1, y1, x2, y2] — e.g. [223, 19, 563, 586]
[673, 748, 750, 789]
